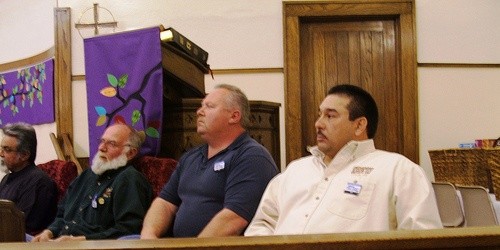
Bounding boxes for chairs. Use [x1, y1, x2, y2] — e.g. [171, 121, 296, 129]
[456, 183, 498, 228]
[431, 181, 466, 229]
[137, 156, 180, 199]
[36, 159, 77, 207]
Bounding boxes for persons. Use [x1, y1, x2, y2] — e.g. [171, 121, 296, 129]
[0, 121, 59, 242]
[116, 84, 281, 240]
[244, 84, 444, 237]
[31, 123, 152, 242]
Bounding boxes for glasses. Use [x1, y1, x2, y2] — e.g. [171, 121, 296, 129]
[5, 147, 21, 153]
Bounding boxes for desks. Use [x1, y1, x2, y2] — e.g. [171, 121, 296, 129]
[0, 225, 500, 250]
[455, 189, 500, 225]
